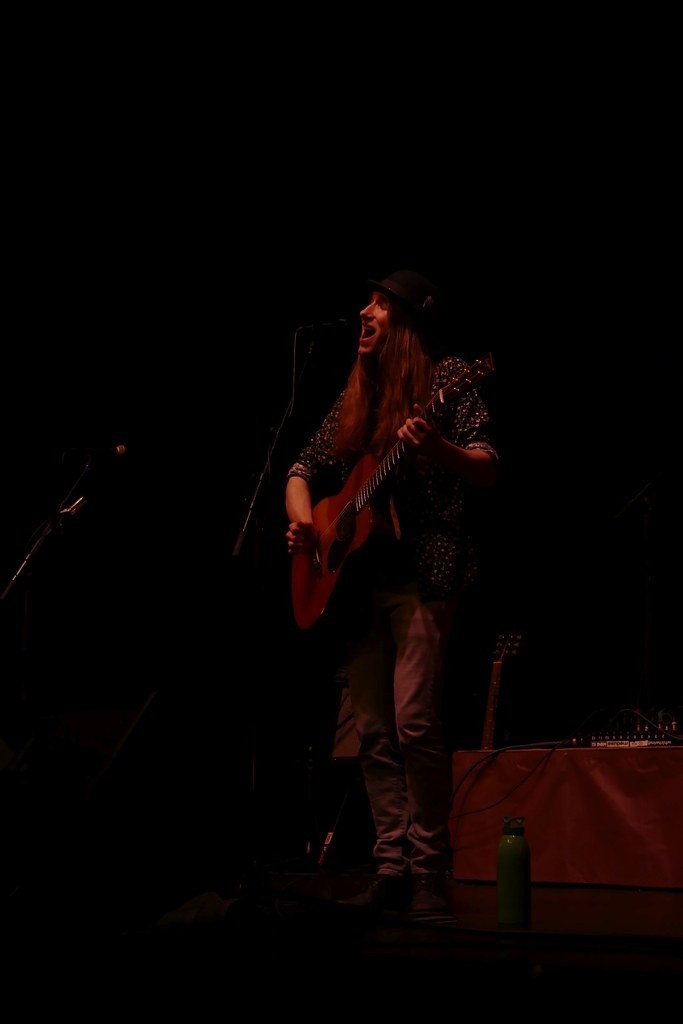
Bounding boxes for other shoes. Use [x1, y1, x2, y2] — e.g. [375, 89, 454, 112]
[406, 873, 457, 923]
[337, 875, 409, 910]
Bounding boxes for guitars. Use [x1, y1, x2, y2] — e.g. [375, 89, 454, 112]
[292, 349, 500, 638]
[480, 631, 526, 750]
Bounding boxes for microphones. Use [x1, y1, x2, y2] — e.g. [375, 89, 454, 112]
[73, 445, 128, 456]
[305, 319, 347, 331]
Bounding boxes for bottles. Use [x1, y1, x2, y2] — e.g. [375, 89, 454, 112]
[494, 816, 532, 926]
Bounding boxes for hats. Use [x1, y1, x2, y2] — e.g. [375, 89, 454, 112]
[368, 270, 443, 330]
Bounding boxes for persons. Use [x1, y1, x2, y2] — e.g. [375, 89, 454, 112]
[283, 264, 503, 926]
